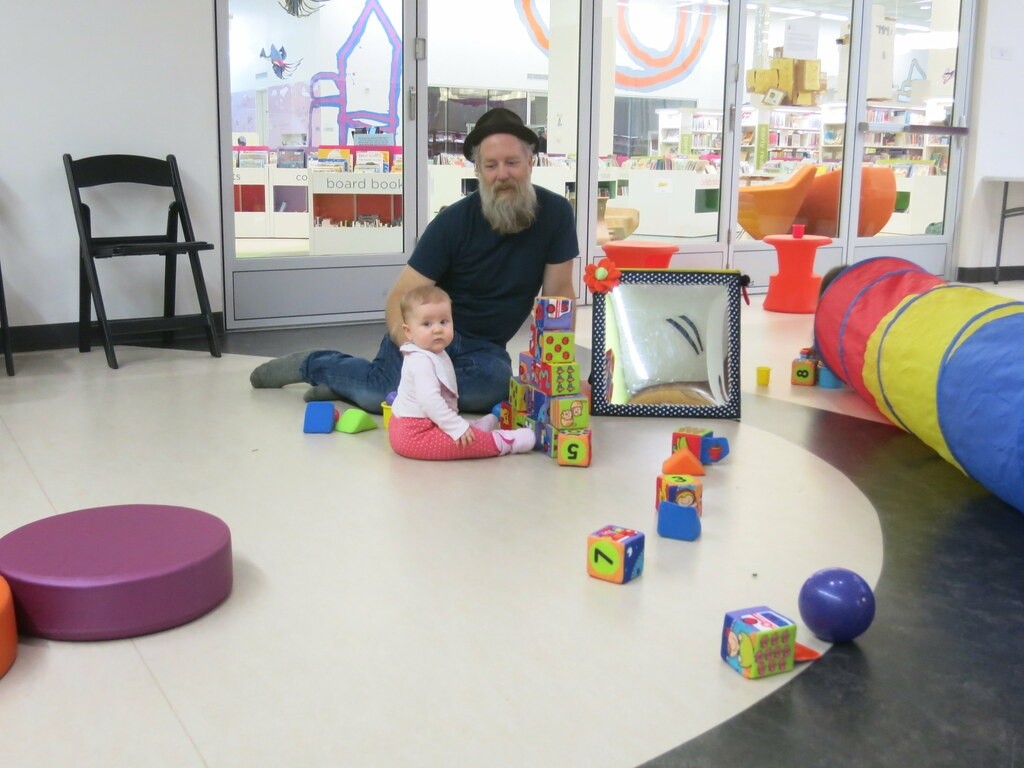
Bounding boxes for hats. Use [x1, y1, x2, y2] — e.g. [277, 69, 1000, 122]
[462, 105, 538, 159]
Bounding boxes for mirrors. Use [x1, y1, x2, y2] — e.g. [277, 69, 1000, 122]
[590, 267, 742, 422]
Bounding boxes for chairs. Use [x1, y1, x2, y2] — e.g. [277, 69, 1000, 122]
[62, 153, 222, 370]
[787, 165, 896, 239]
[736, 163, 817, 240]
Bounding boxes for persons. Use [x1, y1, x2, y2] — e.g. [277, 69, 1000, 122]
[536, 131, 547, 154]
[250, 109, 579, 414]
[389, 286, 537, 462]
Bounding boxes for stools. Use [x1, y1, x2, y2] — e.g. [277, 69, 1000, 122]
[762, 233, 834, 314]
[601, 241, 680, 268]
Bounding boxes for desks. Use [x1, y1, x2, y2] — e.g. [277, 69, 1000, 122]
[983, 175, 1024, 286]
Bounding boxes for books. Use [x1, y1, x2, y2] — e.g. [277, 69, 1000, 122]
[428, 153, 473, 166]
[868, 108, 892, 122]
[231, 149, 402, 173]
[532, 151, 577, 167]
[597, 185, 628, 197]
[760, 112, 844, 176]
[864, 132, 950, 177]
[315, 215, 402, 227]
[740, 111, 756, 175]
[598, 114, 723, 175]
